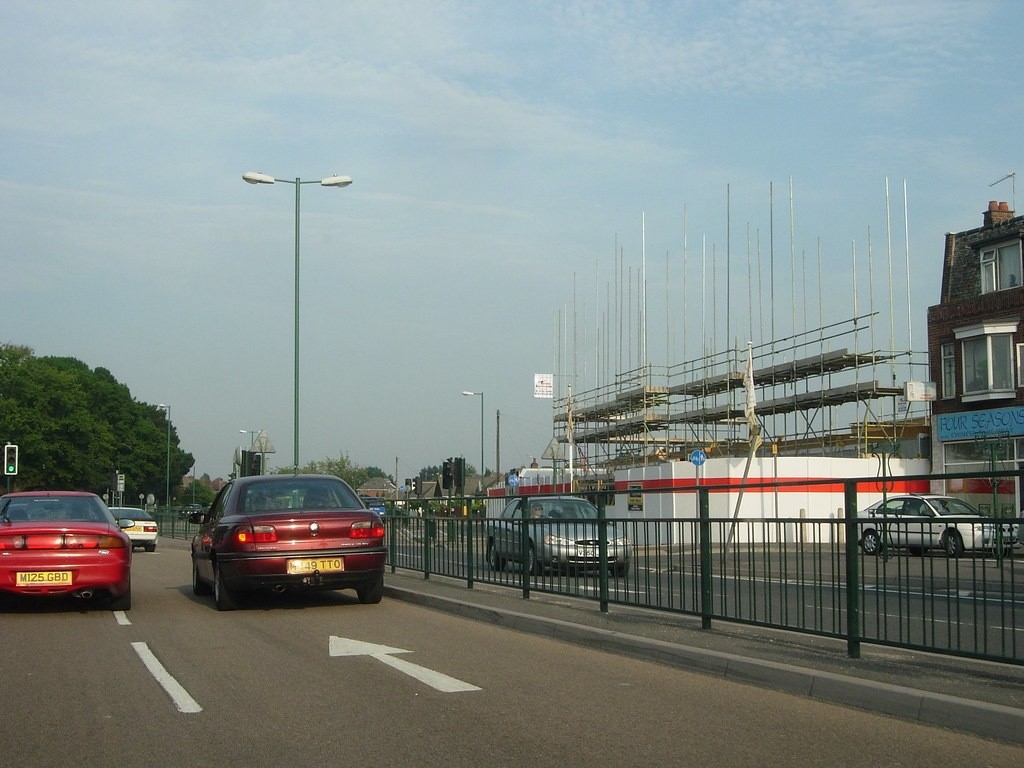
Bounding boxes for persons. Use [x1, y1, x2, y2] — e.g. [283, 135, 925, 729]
[532, 503, 543, 518]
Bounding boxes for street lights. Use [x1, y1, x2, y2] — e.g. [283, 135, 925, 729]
[160, 404, 170, 519]
[239, 430, 261, 446]
[241, 170, 353, 472]
[462, 391, 483, 508]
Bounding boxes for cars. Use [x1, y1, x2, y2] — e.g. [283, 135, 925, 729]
[1017, 510, 1024, 544]
[0, 491, 137, 610]
[858, 492, 1022, 558]
[187, 472, 388, 610]
[486, 495, 631, 578]
[105, 505, 160, 552]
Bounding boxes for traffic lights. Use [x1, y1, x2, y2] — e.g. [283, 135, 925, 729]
[4, 444, 19, 475]
[413, 477, 422, 495]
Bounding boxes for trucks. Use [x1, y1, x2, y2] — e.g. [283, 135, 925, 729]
[361, 497, 387, 524]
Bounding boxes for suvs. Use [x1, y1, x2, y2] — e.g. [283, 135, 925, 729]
[178, 504, 205, 521]
